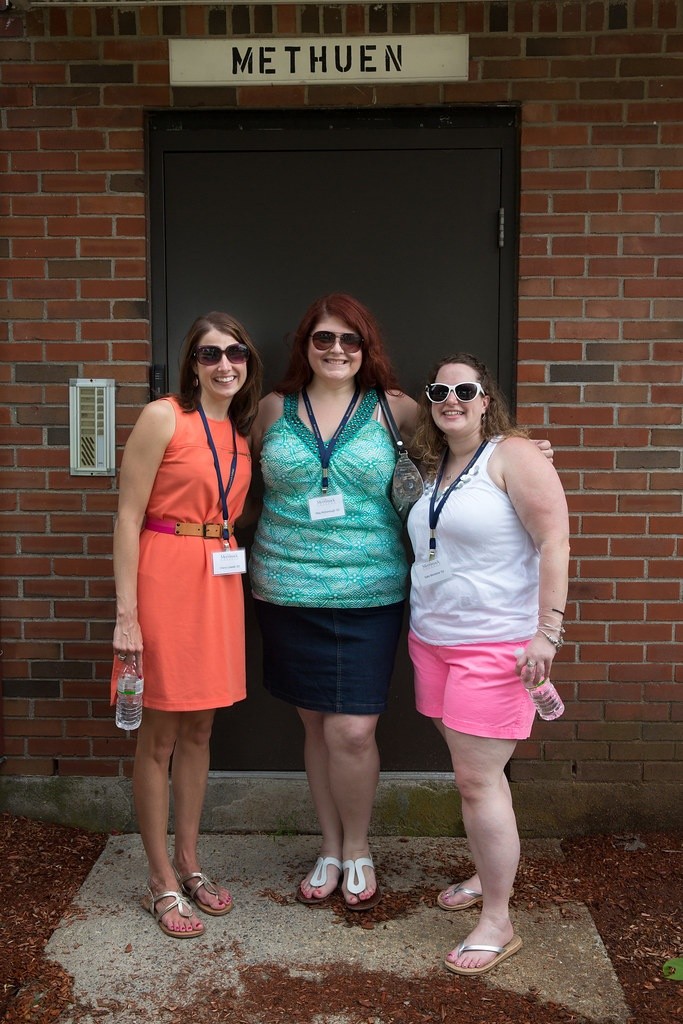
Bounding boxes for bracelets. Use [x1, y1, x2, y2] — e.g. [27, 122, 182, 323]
[537, 609, 567, 652]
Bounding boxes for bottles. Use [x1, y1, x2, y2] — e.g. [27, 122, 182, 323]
[513, 648, 566, 721]
[115, 655, 145, 731]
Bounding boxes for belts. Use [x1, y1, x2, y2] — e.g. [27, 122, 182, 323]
[145, 518, 234, 539]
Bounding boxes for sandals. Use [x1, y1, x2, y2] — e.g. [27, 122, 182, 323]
[138, 876, 206, 938]
[170, 858, 234, 916]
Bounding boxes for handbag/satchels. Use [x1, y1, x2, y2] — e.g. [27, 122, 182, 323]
[373, 383, 425, 523]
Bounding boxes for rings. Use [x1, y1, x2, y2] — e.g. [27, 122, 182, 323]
[117, 650, 127, 662]
[525, 659, 537, 669]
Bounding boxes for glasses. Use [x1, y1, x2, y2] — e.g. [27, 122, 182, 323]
[308, 330, 365, 353]
[192, 342, 250, 366]
[423, 382, 485, 404]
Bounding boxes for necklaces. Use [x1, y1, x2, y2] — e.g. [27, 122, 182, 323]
[444, 448, 475, 480]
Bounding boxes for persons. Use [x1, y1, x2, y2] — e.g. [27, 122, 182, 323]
[241, 292, 433, 911]
[109, 310, 264, 940]
[407, 350, 572, 978]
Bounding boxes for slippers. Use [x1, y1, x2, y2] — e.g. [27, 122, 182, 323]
[444, 933, 523, 976]
[436, 880, 515, 911]
[296, 857, 343, 904]
[341, 850, 382, 910]
